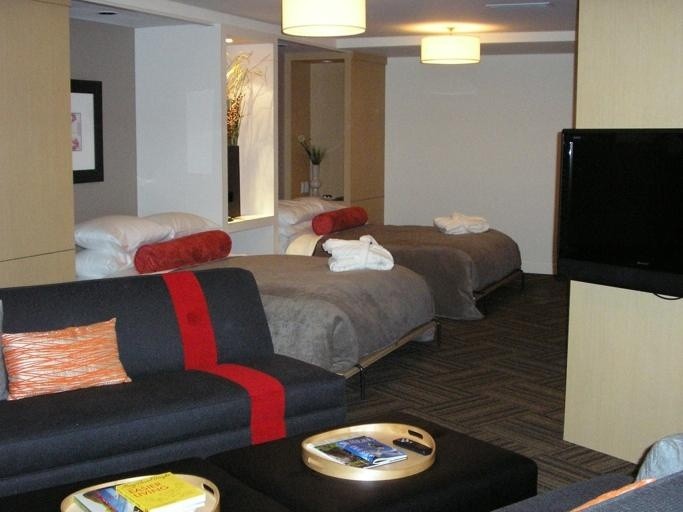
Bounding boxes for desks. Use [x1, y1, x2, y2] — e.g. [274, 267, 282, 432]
[0, 454, 294, 512]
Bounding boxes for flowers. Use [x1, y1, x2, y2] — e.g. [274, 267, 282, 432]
[296, 130, 330, 165]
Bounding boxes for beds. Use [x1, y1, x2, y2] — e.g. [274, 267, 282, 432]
[165, 252, 440, 399]
[284, 222, 525, 321]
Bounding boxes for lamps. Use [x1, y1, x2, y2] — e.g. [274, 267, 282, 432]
[418, 26, 482, 66]
[279, 0, 367, 39]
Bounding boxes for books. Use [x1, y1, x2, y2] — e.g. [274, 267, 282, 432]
[336, 434, 407, 464]
[114, 469, 207, 512]
[72, 483, 196, 512]
[307, 434, 406, 469]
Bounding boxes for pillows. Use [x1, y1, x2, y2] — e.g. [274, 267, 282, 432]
[277, 195, 369, 236]
[142, 211, 221, 241]
[0, 317, 133, 403]
[69, 208, 169, 253]
[134, 229, 232, 275]
[74, 249, 141, 280]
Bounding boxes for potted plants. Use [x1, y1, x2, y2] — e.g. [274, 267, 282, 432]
[223, 47, 267, 147]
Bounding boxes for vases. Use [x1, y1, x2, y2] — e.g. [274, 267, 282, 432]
[311, 164, 320, 198]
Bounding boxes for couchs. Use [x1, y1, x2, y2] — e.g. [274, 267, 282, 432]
[488, 433, 682, 511]
[0, 266, 349, 504]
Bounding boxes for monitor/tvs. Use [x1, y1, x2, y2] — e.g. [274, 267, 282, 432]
[556, 128, 683, 298]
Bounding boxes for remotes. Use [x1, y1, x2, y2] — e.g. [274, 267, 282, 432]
[393, 438, 432, 456]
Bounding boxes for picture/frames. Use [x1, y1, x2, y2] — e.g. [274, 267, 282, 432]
[69, 79, 105, 184]
